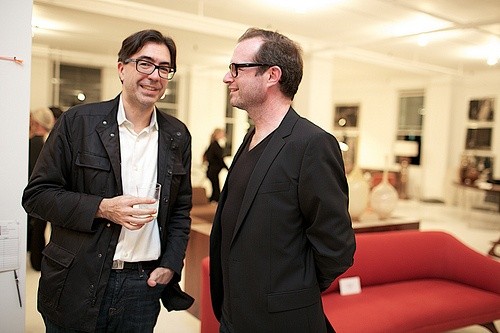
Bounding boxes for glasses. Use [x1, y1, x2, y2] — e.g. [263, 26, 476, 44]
[124, 59, 176, 81]
[229, 63, 271, 78]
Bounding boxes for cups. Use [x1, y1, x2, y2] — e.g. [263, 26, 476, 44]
[136, 182, 161, 219]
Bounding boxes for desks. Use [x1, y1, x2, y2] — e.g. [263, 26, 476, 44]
[184, 211, 420, 322]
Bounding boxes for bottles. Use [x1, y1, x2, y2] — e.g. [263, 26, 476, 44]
[347, 145, 368, 219]
[371, 158, 397, 220]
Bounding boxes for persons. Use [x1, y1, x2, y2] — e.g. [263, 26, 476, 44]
[22, 30, 196, 333]
[210, 28, 356, 333]
[27, 106, 63, 271]
[204, 128, 229, 202]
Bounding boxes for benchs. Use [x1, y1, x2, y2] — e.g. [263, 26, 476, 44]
[201, 232, 500, 333]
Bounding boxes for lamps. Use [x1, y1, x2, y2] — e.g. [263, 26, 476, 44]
[395, 140, 418, 201]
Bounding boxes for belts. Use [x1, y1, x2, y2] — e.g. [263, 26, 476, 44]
[112, 259, 151, 271]
